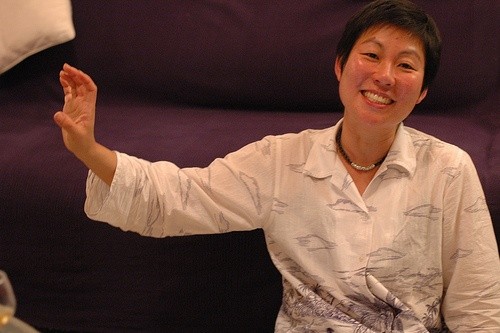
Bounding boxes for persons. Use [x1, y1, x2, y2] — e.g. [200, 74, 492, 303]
[52, 0, 500, 333]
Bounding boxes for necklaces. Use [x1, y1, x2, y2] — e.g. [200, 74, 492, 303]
[336, 126, 390, 173]
